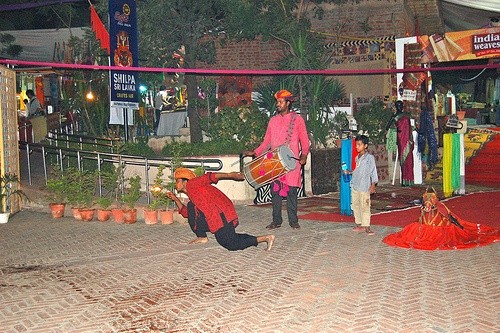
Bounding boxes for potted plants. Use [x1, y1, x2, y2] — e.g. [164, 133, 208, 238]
[39, 161, 183, 226]
[0, 173, 31, 224]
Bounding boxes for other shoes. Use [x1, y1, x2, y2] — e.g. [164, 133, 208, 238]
[353, 225, 362, 231]
[366, 229, 374, 235]
[267, 223, 280, 229]
[291, 224, 300, 230]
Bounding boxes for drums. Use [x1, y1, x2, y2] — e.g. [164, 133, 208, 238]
[243, 145, 296, 190]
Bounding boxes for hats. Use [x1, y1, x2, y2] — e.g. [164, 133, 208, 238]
[173, 168, 196, 180]
[274, 90, 295, 105]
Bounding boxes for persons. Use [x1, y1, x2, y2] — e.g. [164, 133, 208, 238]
[248, 89, 310, 230]
[387, 100, 415, 187]
[154, 86, 172, 131]
[22, 87, 42, 116]
[165, 168, 275, 251]
[342, 135, 379, 236]
